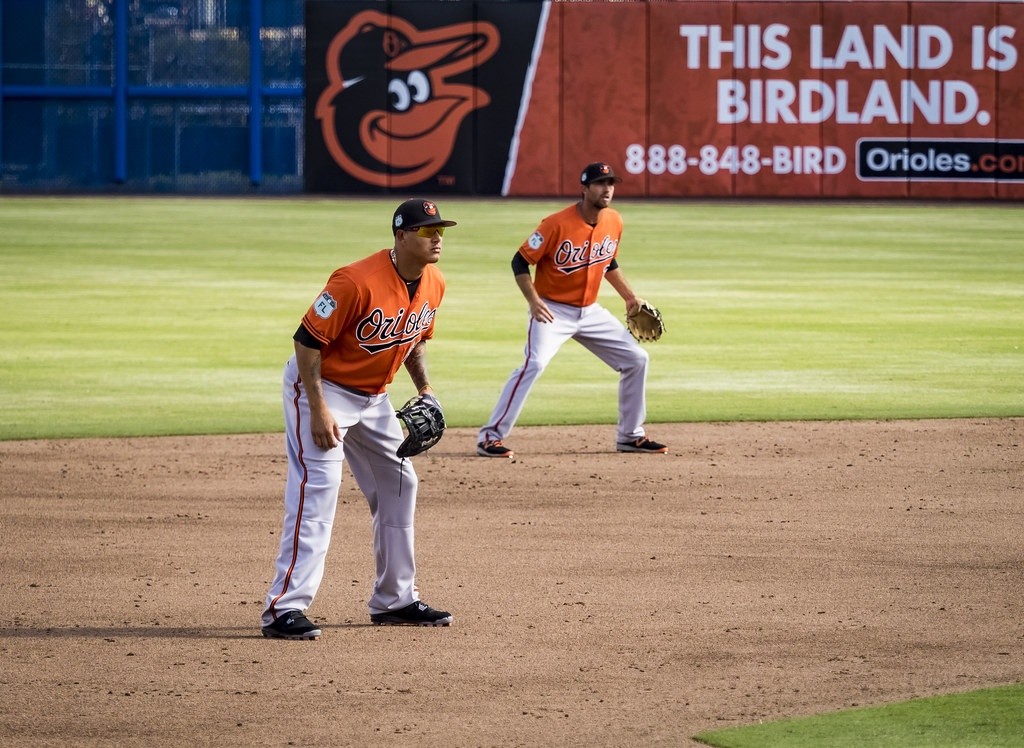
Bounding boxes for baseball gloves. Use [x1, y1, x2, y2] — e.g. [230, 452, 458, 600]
[624, 301, 667, 346]
[393, 393, 449, 461]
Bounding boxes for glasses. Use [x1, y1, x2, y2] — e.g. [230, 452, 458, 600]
[405, 224, 445, 238]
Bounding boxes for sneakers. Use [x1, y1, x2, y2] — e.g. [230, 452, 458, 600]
[616, 436, 668, 453]
[262, 611, 321, 640]
[371, 601, 453, 626]
[477, 440, 514, 456]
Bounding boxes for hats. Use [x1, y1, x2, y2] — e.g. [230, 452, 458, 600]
[392, 198, 457, 232]
[581, 163, 622, 185]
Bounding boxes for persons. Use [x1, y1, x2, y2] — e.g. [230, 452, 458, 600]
[476, 162, 668, 458]
[259, 198, 457, 639]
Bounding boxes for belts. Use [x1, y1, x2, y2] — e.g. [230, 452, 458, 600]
[332, 382, 377, 397]
